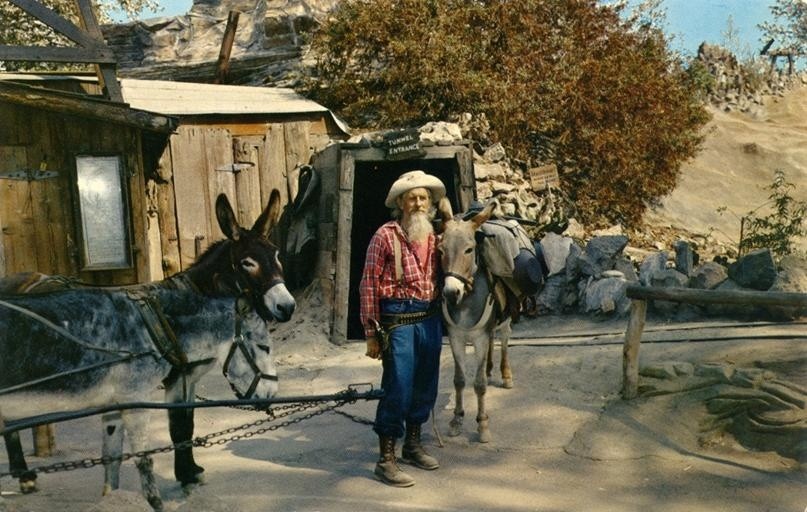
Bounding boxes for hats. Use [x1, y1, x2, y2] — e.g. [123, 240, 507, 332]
[385, 169, 446, 209]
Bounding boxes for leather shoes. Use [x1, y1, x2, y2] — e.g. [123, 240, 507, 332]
[400, 445, 440, 470]
[373, 460, 416, 487]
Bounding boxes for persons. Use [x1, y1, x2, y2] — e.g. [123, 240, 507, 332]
[357, 168, 447, 488]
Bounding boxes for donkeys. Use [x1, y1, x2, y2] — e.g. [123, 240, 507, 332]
[435, 195, 514, 443]
[1, 292, 279, 512]
[0, 189, 298, 496]
